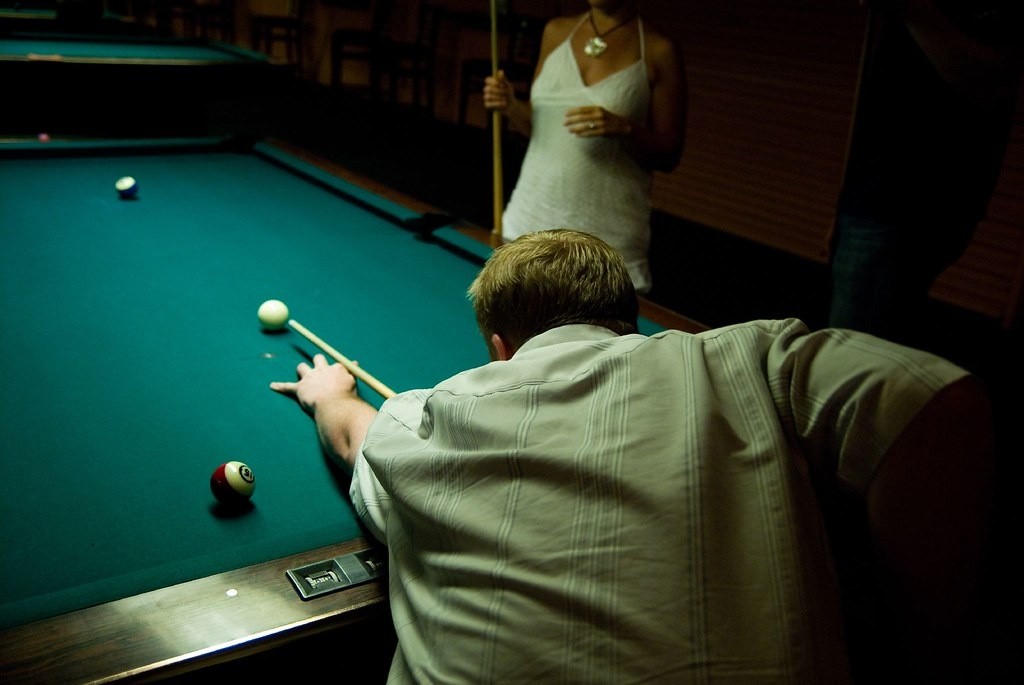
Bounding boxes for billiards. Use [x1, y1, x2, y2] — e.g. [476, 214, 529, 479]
[38, 132, 50, 143]
[115, 175, 138, 198]
[210, 459, 258, 507]
[256, 298, 290, 332]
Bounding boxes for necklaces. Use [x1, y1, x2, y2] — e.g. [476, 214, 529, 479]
[584, 7, 633, 57]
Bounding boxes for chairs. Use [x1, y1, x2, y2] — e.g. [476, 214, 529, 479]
[106, 0, 547, 133]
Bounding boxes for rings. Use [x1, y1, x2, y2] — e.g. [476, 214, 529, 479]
[588, 120, 596, 129]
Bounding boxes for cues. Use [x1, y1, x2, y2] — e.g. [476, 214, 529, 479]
[489, 0, 506, 236]
[287, 318, 400, 402]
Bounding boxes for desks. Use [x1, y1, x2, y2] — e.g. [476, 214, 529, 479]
[0, 137, 714, 685]
[0, 31, 270, 138]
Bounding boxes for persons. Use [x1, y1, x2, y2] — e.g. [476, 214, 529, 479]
[482, 0, 689, 295]
[270, 226, 978, 685]
